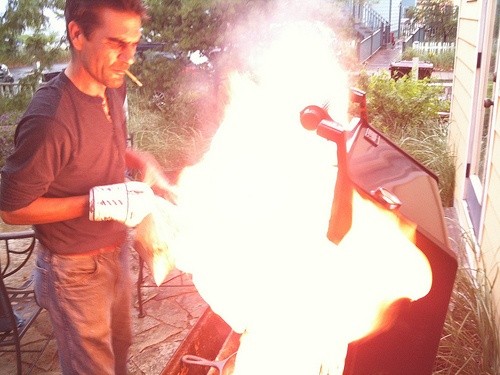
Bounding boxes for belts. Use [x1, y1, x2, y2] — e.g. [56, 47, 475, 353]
[63, 243, 123, 254]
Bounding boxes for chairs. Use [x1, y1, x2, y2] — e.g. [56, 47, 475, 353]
[0, 231, 196, 375]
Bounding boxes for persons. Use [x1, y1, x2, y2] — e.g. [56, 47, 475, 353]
[0, 0, 160, 375]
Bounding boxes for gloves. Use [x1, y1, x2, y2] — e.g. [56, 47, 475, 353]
[89, 182, 153, 227]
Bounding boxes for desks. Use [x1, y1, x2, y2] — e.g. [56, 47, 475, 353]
[390, 60, 435, 81]
